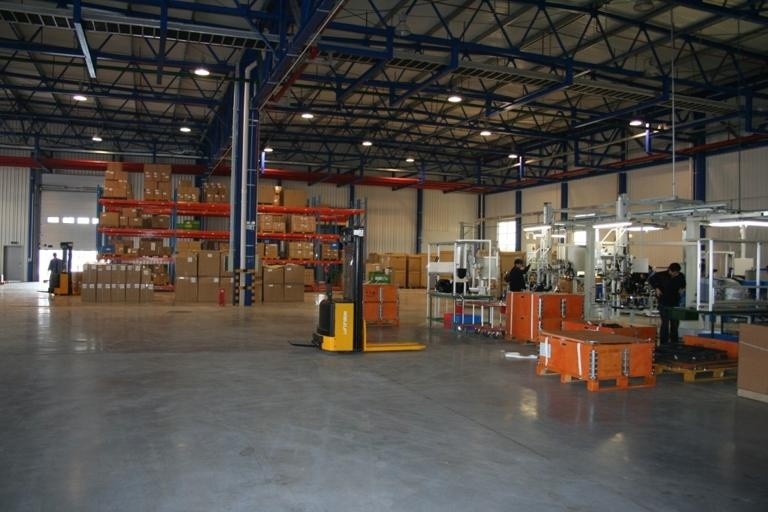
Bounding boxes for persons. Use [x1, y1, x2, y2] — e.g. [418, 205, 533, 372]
[507, 256, 533, 292]
[46, 252, 64, 292]
[648, 261, 686, 341]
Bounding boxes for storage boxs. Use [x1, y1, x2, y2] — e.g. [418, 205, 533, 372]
[737, 322, 768, 404]
[79, 160, 340, 303]
[366, 249, 526, 290]
